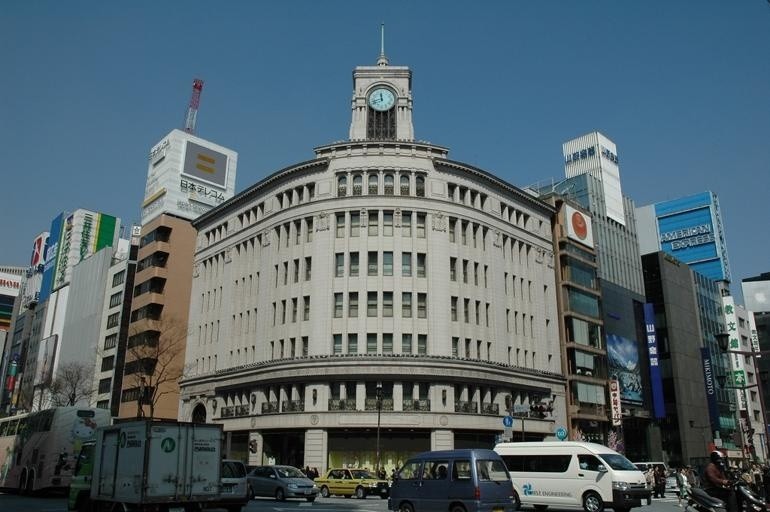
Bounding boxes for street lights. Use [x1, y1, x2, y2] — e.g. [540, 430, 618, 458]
[375, 380, 384, 466]
[714, 327, 769, 453]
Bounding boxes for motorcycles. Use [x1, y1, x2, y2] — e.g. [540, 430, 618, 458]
[685, 479, 768, 512]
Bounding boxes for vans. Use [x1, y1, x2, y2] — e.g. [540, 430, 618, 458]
[388, 441, 651, 512]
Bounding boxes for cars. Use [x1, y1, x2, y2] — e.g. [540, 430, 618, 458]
[633, 461, 700, 493]
[223, 458, 389, 512]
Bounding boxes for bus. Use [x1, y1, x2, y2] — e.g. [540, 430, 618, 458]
[0, 406, 111, 497]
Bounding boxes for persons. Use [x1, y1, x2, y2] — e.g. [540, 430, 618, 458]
[645, 466, 695, 507]
[701, 450, 741, 512]
[314, 468, 319, 477]
[307, 468, 314, 480]
[436, 465, 448, 481]
[580, 458, 588, 469]
[378, 467, 386, 480]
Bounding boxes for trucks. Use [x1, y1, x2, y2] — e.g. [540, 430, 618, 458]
[67, 420, 223, 511]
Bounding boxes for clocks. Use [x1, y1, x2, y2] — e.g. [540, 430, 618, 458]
[368, 88, 396, 111]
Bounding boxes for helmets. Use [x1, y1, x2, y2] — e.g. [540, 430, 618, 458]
[711, 450, 726, 463]
